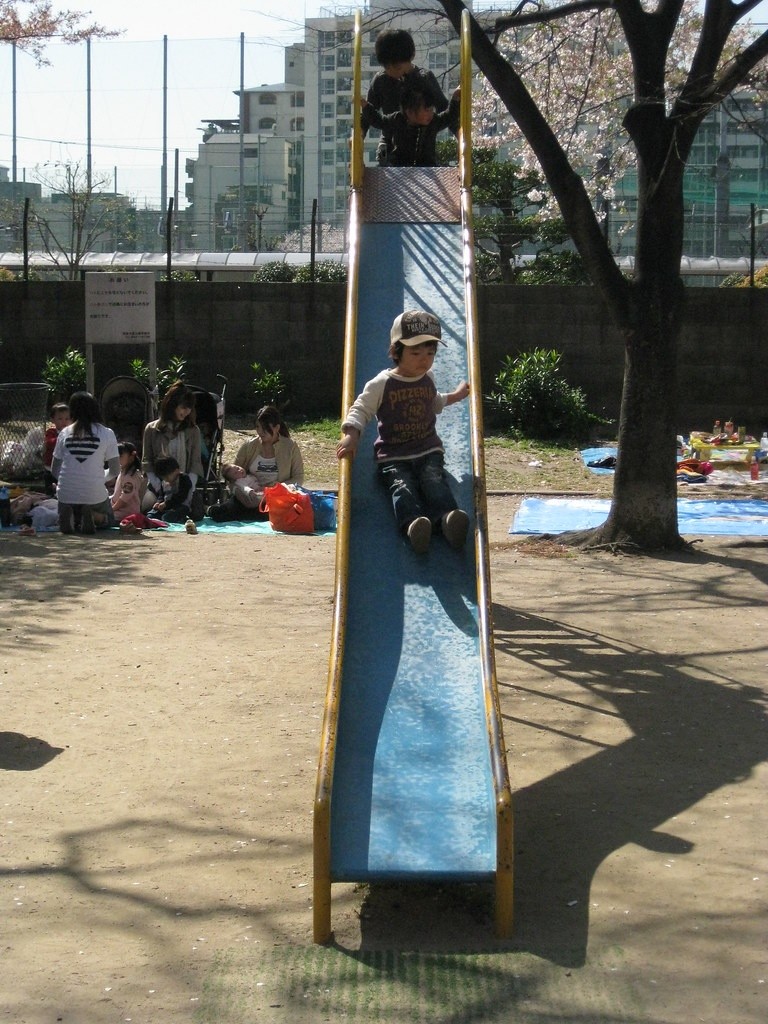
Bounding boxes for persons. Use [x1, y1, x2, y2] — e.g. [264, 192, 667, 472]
[140, 377, 202, 512]
[350, 28, 460, 166]
[51, 391, 121, 535]
[335, 309, 469, 554]
[43, 402, 73, 497]
[0, 495, 59, 536]
[220, 464, 266, 508]
[109, 441, 146, 521]
[203, 405, 305, 521]
[350, 83, 462, 167]
[146, 456, 204, 524]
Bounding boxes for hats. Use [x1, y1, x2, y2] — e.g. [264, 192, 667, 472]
[387, 310, 449, 351]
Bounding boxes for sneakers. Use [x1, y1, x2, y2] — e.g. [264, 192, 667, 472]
[405, 516, 431, 554]
[439, 508, 471, 550]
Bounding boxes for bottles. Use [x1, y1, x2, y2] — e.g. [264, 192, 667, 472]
[724, 422, 733, 438]
[749, 456, 759, 480]
[713, 421, 721, 440]
[761, 432, 768, 453]
[738, 427, 745, 444]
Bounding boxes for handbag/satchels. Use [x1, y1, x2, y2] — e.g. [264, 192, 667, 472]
[258, 482, 316, 536]
[295, 483, 336, 533]
[9, 494, 33, 526]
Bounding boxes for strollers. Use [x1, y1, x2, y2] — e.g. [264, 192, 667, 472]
[96, 375, 156, 510]
[154, 374, 230, 525]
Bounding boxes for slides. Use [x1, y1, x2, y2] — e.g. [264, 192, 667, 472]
[306, 9, 520, 944]
